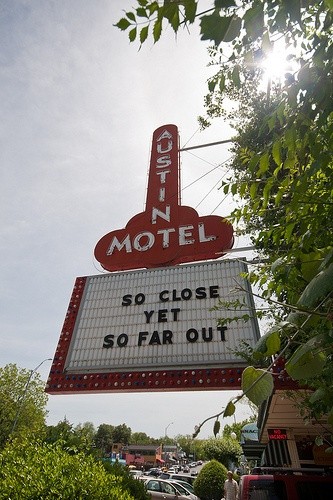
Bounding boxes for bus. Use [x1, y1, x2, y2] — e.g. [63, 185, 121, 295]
[237, 466, 333, 500]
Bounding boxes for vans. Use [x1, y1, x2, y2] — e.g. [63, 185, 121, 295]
[157, 473, 197, 486]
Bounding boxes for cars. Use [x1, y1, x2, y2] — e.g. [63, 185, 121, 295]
[139, 478, 201, 500]
[126, 459, 202, 483]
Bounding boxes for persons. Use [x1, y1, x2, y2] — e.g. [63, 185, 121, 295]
[224, 470, 240, 500]
